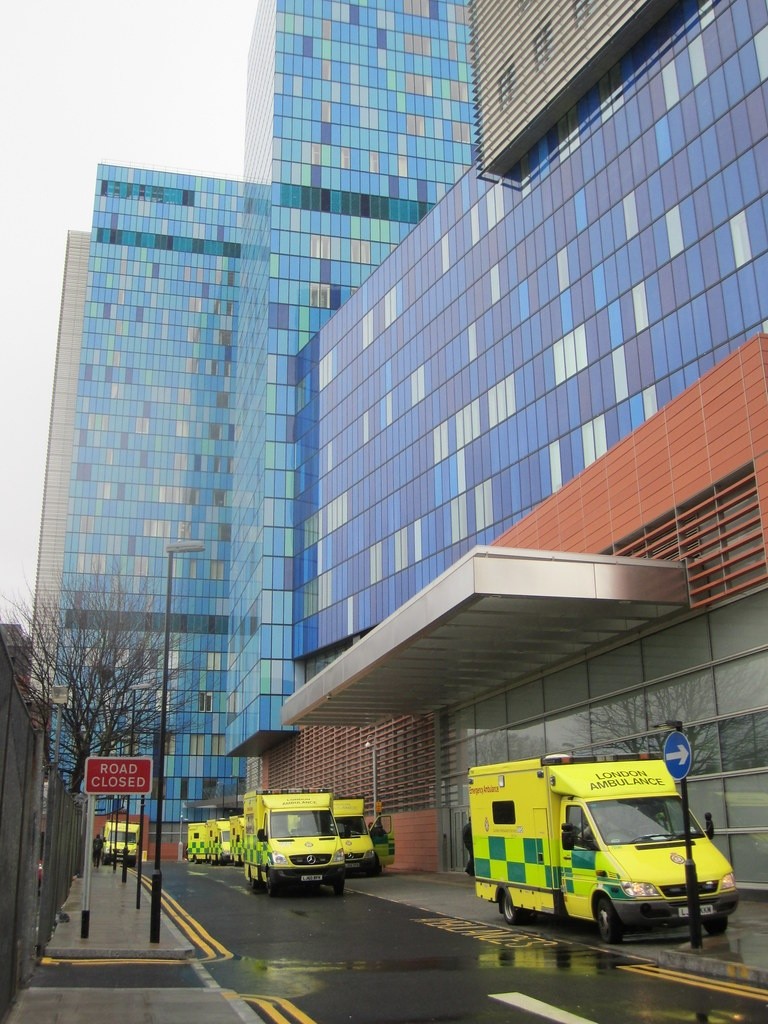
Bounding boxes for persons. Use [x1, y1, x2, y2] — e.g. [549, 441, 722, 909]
[93, 834, 103, 867]
[577, 810, 608, 849]
[368, 822, 373, 828]
[463, 817, 475, 876]
[185, 843, 188, 860]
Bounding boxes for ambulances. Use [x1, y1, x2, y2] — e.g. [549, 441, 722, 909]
[229, 815, 245, 867]
[102, 821, 140, 868]
[332, 797, 396, 878]
[467, 752, 739, 944]
[188, 818, 233, 866]
[244, 788, 345, 895]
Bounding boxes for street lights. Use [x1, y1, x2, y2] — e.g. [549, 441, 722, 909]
[365, 734, 377, 822]
[151, 543, 206, 944]
[121, 683, 153, 882]
